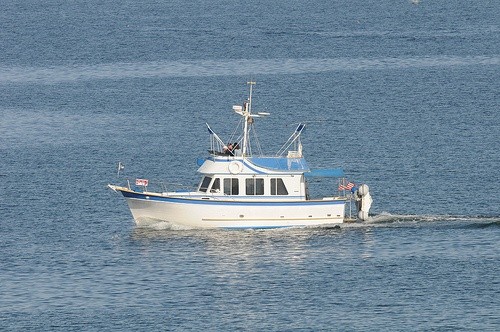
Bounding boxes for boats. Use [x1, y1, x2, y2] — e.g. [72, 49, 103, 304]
[107, 81, 348, 229]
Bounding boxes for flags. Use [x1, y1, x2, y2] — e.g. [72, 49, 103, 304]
[338, 178, 354, 192]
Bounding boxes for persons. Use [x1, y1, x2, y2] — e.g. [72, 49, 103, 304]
[227, 143, 234, 157]
[233, 142, 239, 149]
